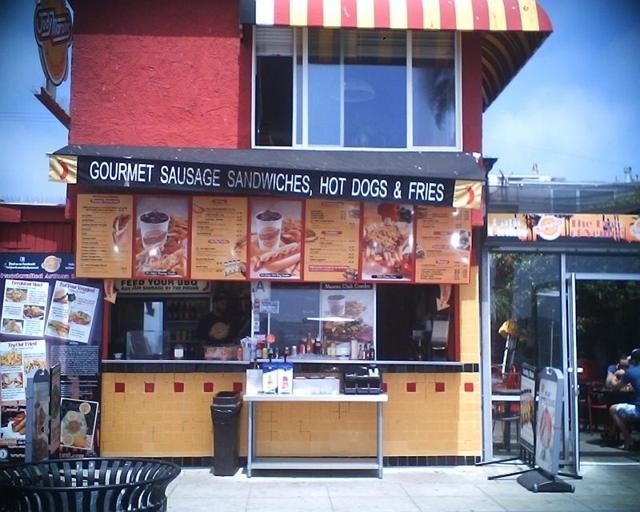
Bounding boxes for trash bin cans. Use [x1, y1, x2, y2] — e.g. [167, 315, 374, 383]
[211, 391, 243, 476]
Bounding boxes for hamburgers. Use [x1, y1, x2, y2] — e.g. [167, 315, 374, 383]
[54, 287, 68, 303]
[143, 230, 166, 248]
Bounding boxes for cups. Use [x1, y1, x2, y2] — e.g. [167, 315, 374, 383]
[255, 211, 283, 252]
[246, 368, 262, 396]
[327, 295, 345, 317]
[137, 210, 170, 253]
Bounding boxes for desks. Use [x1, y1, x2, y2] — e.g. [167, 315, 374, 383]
[241, 390, 390, 480]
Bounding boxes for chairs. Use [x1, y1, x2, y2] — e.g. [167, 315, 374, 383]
[491, 356, 640, 453]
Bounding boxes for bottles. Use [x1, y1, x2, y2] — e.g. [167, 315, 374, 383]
[238, 342, 252, 360]
[168, 299, 207, 358]
[257, 332, 375, 361]
[491, 216, 627, 241]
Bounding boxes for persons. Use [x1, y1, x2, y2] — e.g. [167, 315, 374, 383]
[198, 293, 234, 342]
[609, 347, 640, 450]
[604, 353, 633, 393]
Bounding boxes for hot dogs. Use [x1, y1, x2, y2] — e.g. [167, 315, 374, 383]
[254, 242, 301, 273]
[48, 321, 69, 338]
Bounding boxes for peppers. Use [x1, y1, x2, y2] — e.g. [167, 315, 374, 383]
[378, 202, 404, 222]
[466, 187, 474, 205]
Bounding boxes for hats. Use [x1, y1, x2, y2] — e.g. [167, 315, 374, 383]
[627, 349, 640, 363]
